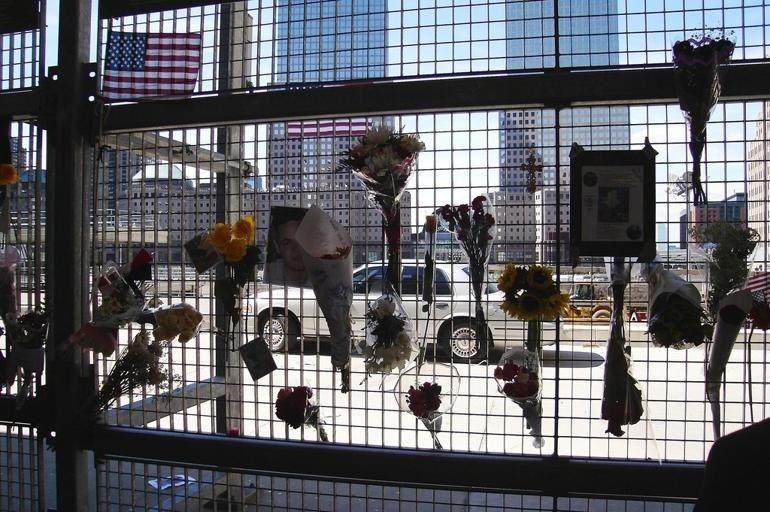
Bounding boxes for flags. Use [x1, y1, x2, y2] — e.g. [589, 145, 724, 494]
[738, 271, 770, 303]
[102, 32, 200, 100]
[286, 81, 373, 136]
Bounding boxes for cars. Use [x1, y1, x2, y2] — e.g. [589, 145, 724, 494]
[243, 258, 564, 364]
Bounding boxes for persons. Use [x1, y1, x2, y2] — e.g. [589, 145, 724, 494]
[265, 219, 315, 289]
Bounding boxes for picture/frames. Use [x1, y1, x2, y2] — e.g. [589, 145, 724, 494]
[569, 137, 657, 270]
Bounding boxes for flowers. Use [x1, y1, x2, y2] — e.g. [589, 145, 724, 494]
[364, 293, 418, 373]
[0, 246, 20, 337]
[673, 38, 736, 207]
[134, 303, 203, 343]
[208, 217, 262, 355]
[46, 331, 166, 454]
[54, 327, 118, 360]
[298, 206, 354, 393]
[706, 287, 753, 441]
[432, 197, 499, 351]
[276, 386, 328, 443]
[406, 383, 443, 451]
[416, 217, 438, 376]
[641, 259, 708, 347]
[1, 165, 18, 248]
[0, 312, 50, 424]
[496, 263, 572, 361]
[94, 261, 138, 307]
[494, 348, 542, 445]
[92, 295, 151, 329]
[93, 249, 153, 290]
[600, 257, 642, 436]
[688, 219, 761, 348]
[748, 299, 770, 424]
[334, 126, 424, 297]
